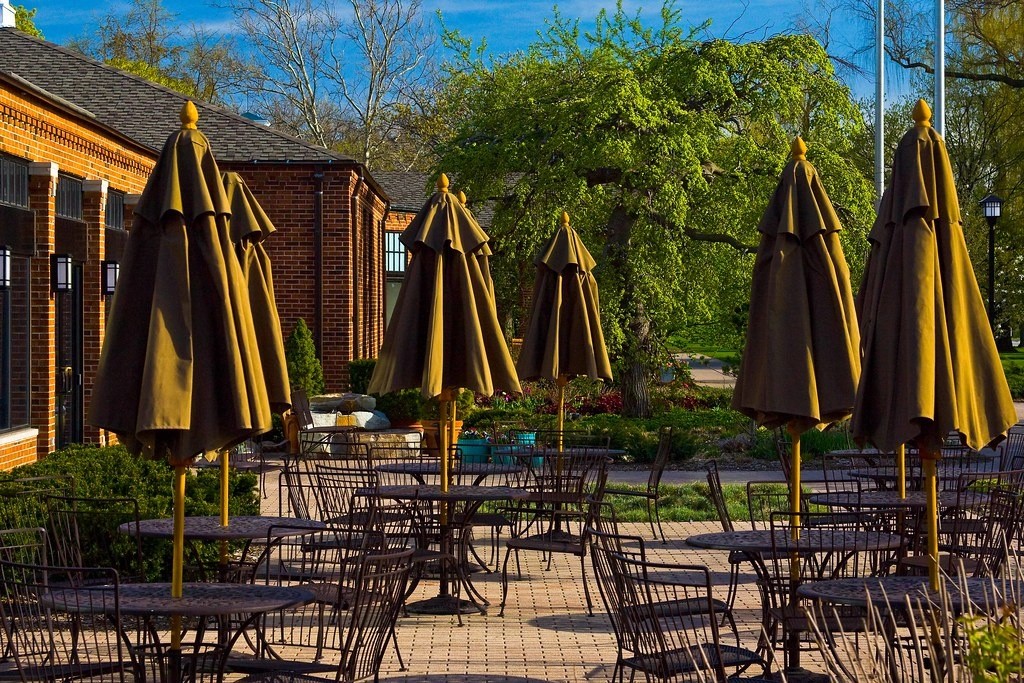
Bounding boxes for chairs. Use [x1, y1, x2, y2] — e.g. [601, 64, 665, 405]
[0, 419, 1024, 683]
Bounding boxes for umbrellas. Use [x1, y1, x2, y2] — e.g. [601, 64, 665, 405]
[853, 94, 1024, 683]
[512, 208, 613, 551]
[724, 132, 872, 683]
[366, 167, 527, 615]
[85, 101, 294, 683]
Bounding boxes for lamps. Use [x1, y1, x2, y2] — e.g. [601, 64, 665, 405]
[1, 244, 12, 290]
[50, 253, 72, 292]
[100, 260, 121, 295]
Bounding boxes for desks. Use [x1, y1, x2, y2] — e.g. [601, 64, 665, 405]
[810, 489, 992, 575]
[120, 515, 325, 664]
[829, 448, 968, 469]
[684, 529, 909, 683]
[374, 460, 523, 575]
[494, 449, 625, 546]
[42, 581, 315, 683]
[355, 485, 530, 616]
[847, 466, 982, 492]
[797, 574, 1024, 683]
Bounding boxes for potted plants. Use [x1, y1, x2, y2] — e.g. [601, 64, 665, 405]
[377, 387, 425, 442]
[421, 387, 476, 459]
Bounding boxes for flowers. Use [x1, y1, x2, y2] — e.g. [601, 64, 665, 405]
[458, 421, 548, 445]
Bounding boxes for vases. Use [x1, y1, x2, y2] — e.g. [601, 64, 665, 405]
[457, 429, 544, 469]
